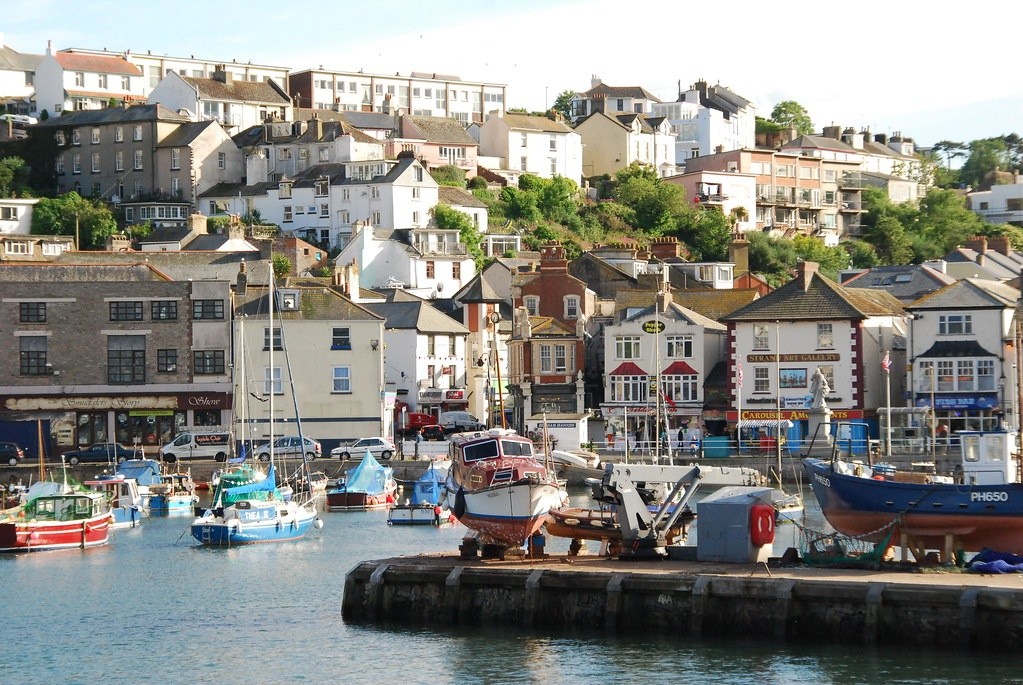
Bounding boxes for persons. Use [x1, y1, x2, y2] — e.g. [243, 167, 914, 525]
[809, 368, 830, 409]
[416, 431, 424, 443]
[632, 422, 767, 458]
[936, 422, 949, 455]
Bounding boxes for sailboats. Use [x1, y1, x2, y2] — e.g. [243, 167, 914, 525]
[634, 301, 686, 505]
[212, 313, 294, 507]
[189, 264, 318, 546]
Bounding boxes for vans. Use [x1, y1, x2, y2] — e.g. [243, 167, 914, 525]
[157, 431, 236, 464]
[439, 412, 487, 435]
[397, 413, 439, 437]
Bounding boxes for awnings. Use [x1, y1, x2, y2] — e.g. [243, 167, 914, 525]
[915, 395, 998, 411]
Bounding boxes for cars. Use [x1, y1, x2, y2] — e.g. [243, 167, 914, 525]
[0, 443, 25, 466]
[330, 437, 396, 461]
[421, 425, 444, 442]
[254, 435, 322, 461]
[60, 442, 143, 465]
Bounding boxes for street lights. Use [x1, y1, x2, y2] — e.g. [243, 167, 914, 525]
[998, 372, 1007, 422]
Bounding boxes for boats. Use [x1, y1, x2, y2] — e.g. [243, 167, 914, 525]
[0, 419, 199, 556]
[798, 421, 1023, 555]
[291, 428, 698, 549]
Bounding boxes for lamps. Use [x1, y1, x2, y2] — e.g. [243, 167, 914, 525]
[45, 363, 54, 374]
[371, 340, 378, 350]
